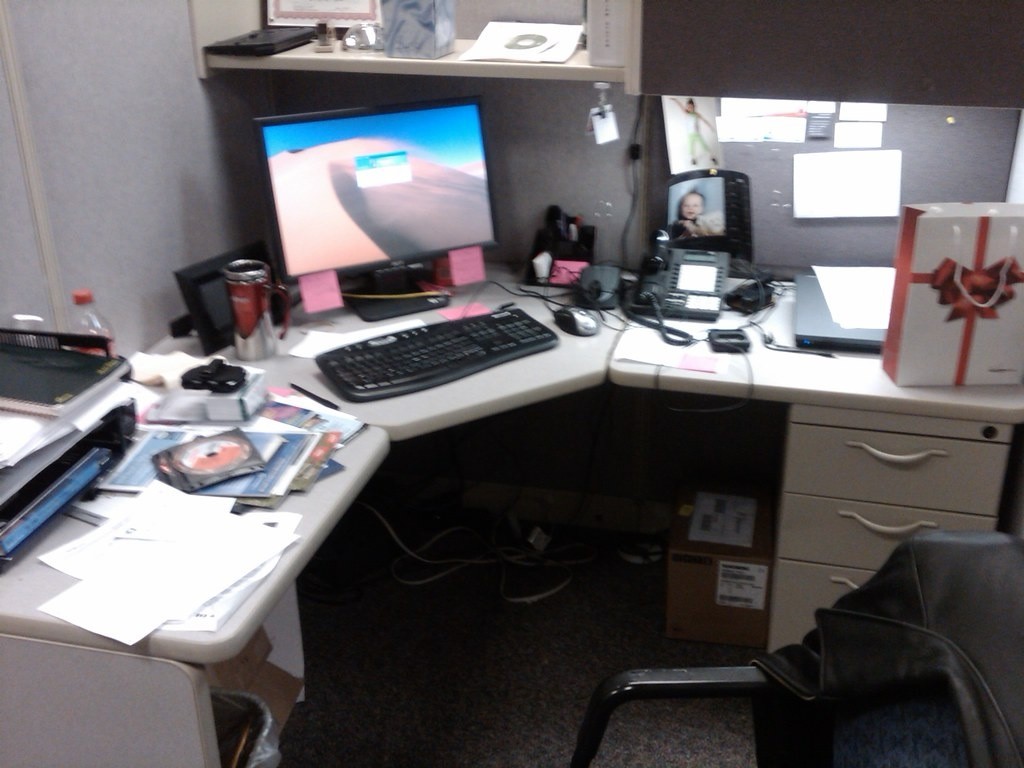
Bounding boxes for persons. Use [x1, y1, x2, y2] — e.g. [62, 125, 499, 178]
[672, 97, 718, 166]
[672, 191, 705, 239]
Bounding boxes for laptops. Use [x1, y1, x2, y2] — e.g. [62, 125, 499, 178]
[793, 273, 889, 353]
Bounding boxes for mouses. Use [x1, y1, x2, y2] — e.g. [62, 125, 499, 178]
[555, 306, 598, 336]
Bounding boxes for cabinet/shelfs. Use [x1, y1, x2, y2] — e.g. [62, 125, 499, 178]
[769, 401, 1013, 654]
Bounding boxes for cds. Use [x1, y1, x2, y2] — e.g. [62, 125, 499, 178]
[505, 33, 547, 50]
[173, 437, 253, 475]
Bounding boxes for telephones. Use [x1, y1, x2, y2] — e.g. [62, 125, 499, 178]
[629, 244, 732, 320]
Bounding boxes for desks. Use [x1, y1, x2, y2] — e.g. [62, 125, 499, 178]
[0, 270, 1024, 768]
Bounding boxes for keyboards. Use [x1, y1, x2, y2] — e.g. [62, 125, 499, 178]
[314, 302, 559, 403]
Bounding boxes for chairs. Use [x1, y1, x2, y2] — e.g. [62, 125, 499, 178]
[564, 529, 1024, 768]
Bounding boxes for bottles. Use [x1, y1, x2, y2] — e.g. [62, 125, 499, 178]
[70, 288, 116, 358]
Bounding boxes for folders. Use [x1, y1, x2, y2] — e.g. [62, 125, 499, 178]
[204, 27, 315, 56]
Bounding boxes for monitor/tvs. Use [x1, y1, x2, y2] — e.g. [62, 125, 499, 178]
[253, 96, 503, 322]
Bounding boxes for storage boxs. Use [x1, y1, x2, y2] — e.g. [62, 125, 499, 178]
[380, 0, 456, 59]
[664, 485, 772, 646]
[879, 200, 1024, 389]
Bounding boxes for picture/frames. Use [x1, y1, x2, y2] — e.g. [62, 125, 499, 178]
[169, 249, 245, 355]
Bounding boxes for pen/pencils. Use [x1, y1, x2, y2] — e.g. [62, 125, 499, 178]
[290, 382, 340, 410]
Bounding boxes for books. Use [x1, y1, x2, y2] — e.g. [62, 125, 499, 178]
[63, 397, 370, 528]
[0, 342, 131, 417]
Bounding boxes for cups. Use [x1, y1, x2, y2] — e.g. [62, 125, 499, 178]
[223, 260, 292, 363]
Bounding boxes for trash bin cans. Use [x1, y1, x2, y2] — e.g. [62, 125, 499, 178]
[210, 686, 273, 768]
